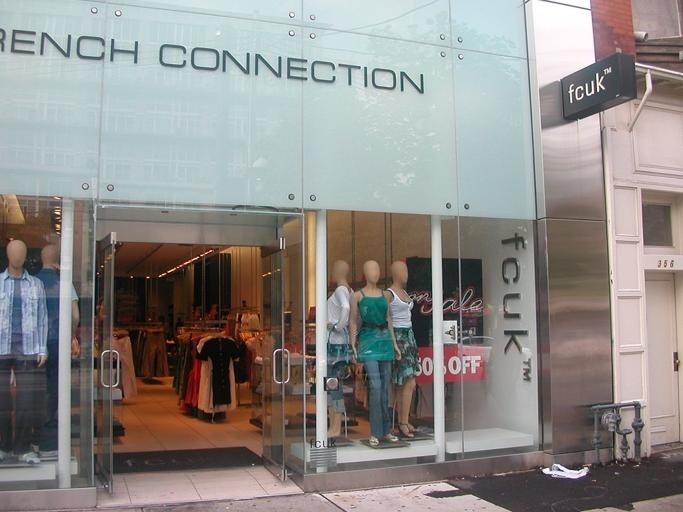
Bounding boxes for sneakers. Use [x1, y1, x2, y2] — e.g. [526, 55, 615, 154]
[370, 437, 379, 446]
[18, 451, 40, 464]
[0, 450, 13, 460]
[31, 444, 58, 457]
[387, 435, 398, 442]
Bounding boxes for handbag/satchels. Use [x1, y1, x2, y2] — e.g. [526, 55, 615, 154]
[327, 356, 357, 394]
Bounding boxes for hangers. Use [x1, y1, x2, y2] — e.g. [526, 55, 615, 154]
[183, 326, 224, 338]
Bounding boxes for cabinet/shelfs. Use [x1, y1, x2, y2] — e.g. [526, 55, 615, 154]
[250, 354, 358, 431]
[72, 358, 124, 440]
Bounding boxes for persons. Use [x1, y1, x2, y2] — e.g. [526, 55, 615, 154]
[349, 260, 401, 447]
[0, 240, 49, 464]
[33, 244, 80, 458]
[386, 260, 418, 438]
[327, 261, 354, 438]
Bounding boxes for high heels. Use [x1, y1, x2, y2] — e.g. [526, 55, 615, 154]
[398, 422, 417, 438]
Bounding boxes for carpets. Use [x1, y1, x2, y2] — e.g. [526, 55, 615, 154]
[94, 446, 272, 475]
